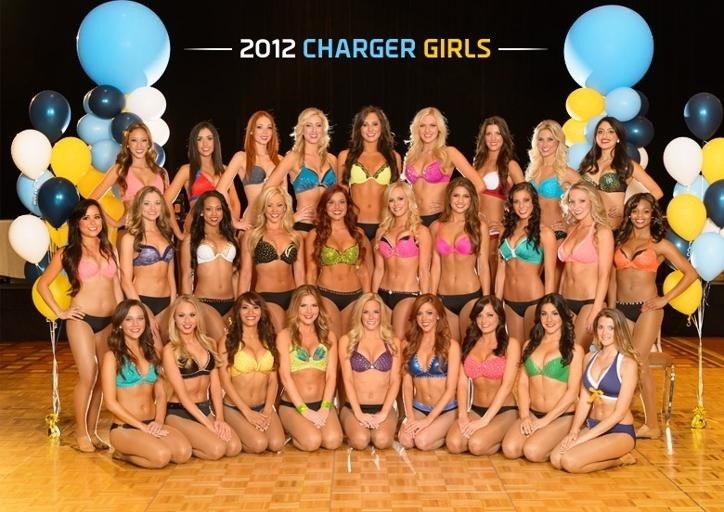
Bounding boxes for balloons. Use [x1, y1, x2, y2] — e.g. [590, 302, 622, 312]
[7, 84, 173, 327]
[562, 4, 655, 93]
[659, 89, 724, 324]
[75, 1, 170, 91]
[559, 90, 657, 188]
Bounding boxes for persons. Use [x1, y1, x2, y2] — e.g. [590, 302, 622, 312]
[35, 105, 699, 477]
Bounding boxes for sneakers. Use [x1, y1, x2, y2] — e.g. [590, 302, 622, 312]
[635, 424, 662, 440]
[77, 432, 111, 453]
